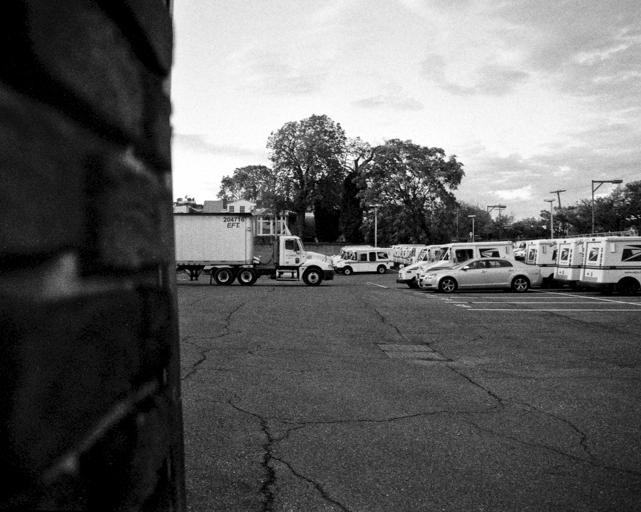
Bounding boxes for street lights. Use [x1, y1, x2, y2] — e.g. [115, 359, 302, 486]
[591, 178, 623, 235]
[487, 205, 507, 239]
[551, 189, 567, 208]
[544, 199, 557, 239]
[467, 214, 476, 241]
[370, 204, 385, 247]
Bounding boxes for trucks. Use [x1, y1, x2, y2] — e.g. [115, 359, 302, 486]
[173, 211, 335, 286]
